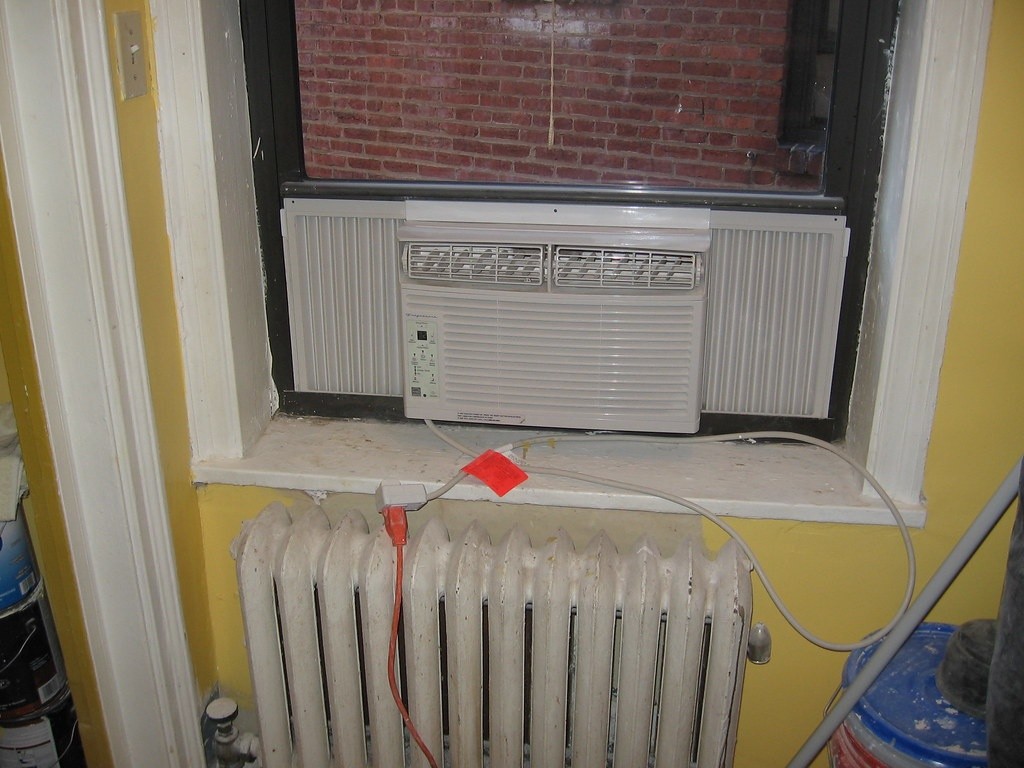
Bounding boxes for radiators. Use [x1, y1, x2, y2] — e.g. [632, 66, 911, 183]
[199, 502, 774, 768]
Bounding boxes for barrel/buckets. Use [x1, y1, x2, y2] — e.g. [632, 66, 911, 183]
[0, 502, 86, 768]
[827, 621, 989, 768]
